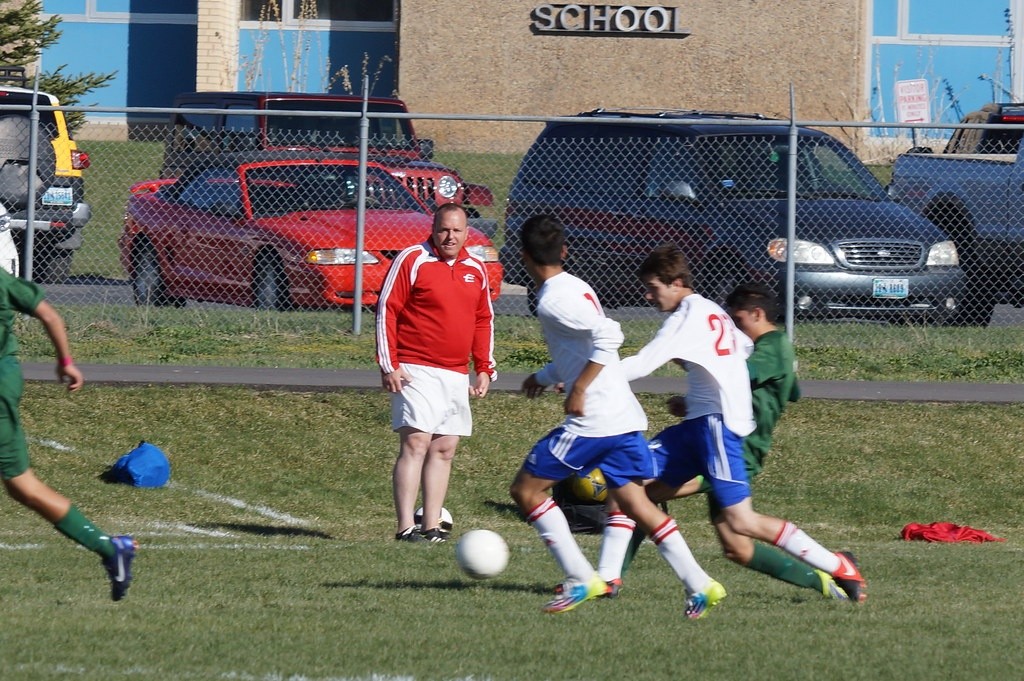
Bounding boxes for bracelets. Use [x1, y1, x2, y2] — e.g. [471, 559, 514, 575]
[59, 356, 73, 365]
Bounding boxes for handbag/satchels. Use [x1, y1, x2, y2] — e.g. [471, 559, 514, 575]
[553, 481, 609, 532]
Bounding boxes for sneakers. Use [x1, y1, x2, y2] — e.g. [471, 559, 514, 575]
[555, 577, 621, 600]
[101, 535, 137, 601]
[542, 572, 611, 614]
[829, 551, 865, 604]
[419, 527, 448, 543]
[685, 580, 728, 620]
[816, 567, 849, 602]
[396, 524, 425, 543]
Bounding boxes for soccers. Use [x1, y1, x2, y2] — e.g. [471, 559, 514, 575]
[456, 529, 510, 580]
[572, 467, 611, 502]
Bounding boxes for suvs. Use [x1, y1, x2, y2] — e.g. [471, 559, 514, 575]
[498, 105, 968, 324]
[159, 90, 500, 241]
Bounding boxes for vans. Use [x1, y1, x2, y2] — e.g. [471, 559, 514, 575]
[0, 85, 91, 284]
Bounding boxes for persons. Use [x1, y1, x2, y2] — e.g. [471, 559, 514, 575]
[508, 214, 868, 620]
[375, 202, 498, 543]
[0, 264, 138, 601]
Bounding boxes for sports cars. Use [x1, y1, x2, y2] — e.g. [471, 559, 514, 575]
[117, 156, 503, 312]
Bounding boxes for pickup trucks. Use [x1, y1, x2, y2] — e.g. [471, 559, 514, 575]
[881, 102, 1023, 307]
